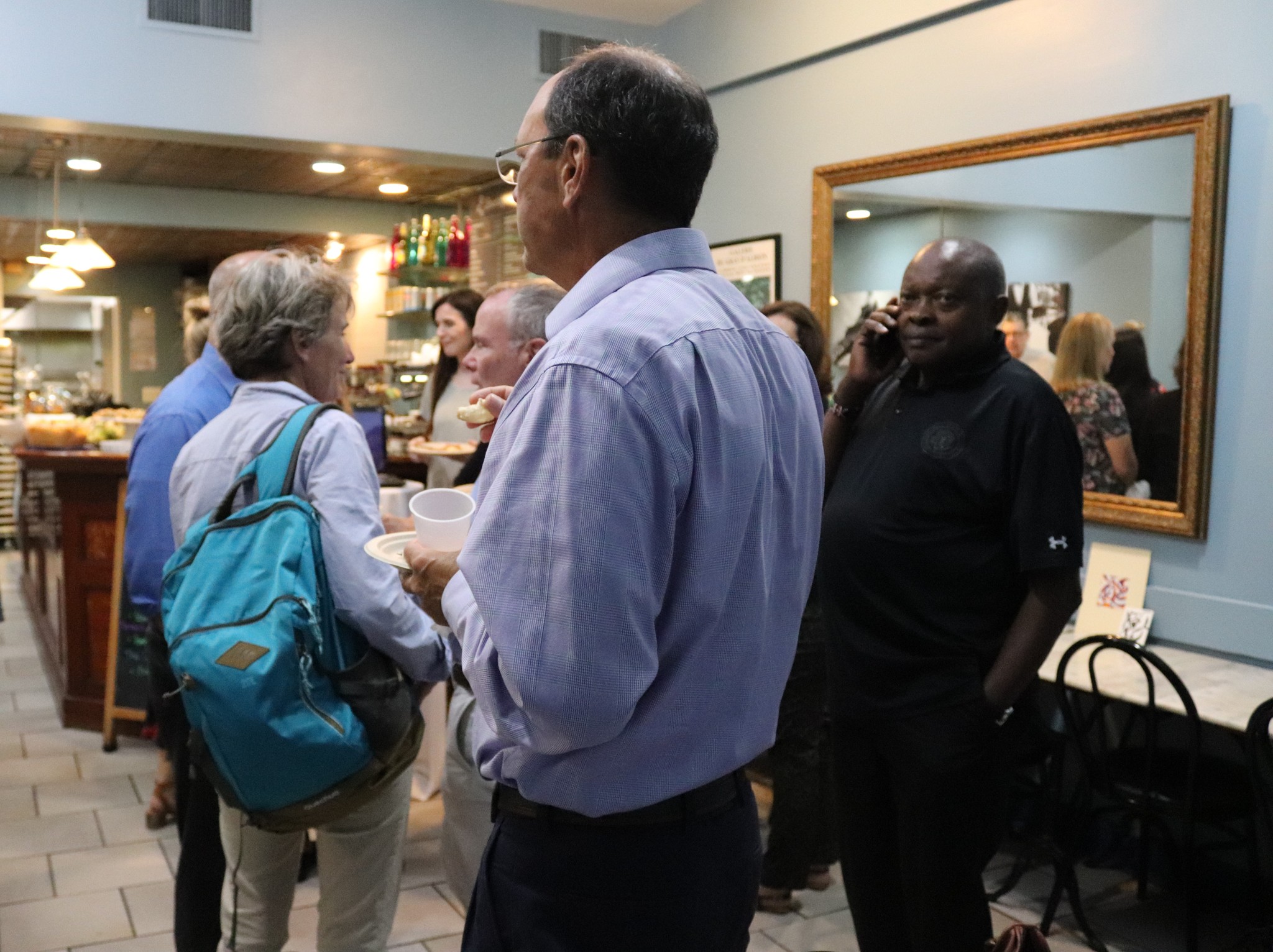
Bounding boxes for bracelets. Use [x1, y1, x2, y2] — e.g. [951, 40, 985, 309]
[829, 402, 856, 420]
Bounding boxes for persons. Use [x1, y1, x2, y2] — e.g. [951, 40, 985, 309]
[1001, 301, 1186, 505]
[747, 299, 836, 909]
[447, 277, 570, 916]
[168, 248, 454, 952]
[145, 296, 215, 827]
[819, 238, 1084, 952]
[413, 289, 487, 492]
[399, 41, 826, 952]
[120, 251, 273, 952]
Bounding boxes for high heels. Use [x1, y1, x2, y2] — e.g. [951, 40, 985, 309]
[146, 778, 179, 828]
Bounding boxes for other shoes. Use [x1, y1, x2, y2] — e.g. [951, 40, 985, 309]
[295, 840, 318, 884]
[756, 887, 801, 912]
[803, 864, 830, 890]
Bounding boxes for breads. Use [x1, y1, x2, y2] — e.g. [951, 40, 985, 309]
[457, 398, 495, 424]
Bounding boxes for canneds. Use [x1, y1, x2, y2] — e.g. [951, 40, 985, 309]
[405, 286, 445, 312]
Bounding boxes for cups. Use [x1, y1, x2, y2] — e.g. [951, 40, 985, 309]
[409, 486, 479, 550]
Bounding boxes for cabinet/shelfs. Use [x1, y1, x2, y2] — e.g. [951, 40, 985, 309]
[378, 269, 470, 322]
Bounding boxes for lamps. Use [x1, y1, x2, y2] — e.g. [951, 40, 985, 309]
[48, 137, 115, 271]
[28, 138, 86, 290]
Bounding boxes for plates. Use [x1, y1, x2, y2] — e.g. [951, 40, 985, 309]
[99, 439, 134, 454]
[407, 443, 476, 454]
[363, 529, 419, 573]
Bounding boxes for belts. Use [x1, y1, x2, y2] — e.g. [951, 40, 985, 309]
[490, 765, 748, 822]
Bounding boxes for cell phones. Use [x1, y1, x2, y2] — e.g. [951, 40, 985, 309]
[873, 315, 898, 368]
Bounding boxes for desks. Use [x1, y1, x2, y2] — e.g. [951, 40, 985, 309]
[1040, 630, 1273, 952]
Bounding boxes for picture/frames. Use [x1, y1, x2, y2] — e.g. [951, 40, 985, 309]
[709, 233, 782, 311]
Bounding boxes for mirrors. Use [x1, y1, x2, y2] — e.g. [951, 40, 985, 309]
[810, 95, 1231, 543]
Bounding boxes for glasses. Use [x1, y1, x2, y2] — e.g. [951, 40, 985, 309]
[494, 133, 570, 186]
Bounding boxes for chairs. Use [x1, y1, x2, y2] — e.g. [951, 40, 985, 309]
[980, 636, 1273, 952]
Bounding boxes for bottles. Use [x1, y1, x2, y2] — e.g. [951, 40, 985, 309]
[391, 215, 476, 284]
[354, 358, 441, 396]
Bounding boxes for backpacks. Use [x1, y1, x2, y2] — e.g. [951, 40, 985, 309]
[161, 400, 426, 836]
[986, 924, 1051, 952]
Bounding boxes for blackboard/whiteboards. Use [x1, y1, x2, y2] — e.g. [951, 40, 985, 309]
[101, 478, 156, 722]
